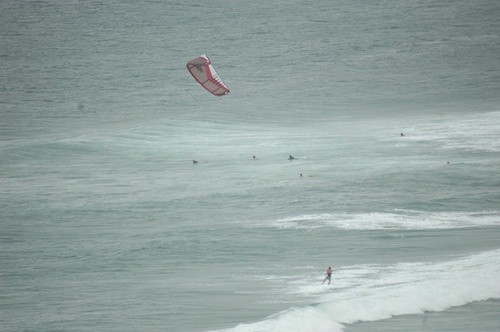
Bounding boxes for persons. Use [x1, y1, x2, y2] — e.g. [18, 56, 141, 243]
[321, 267, 334, 285]
